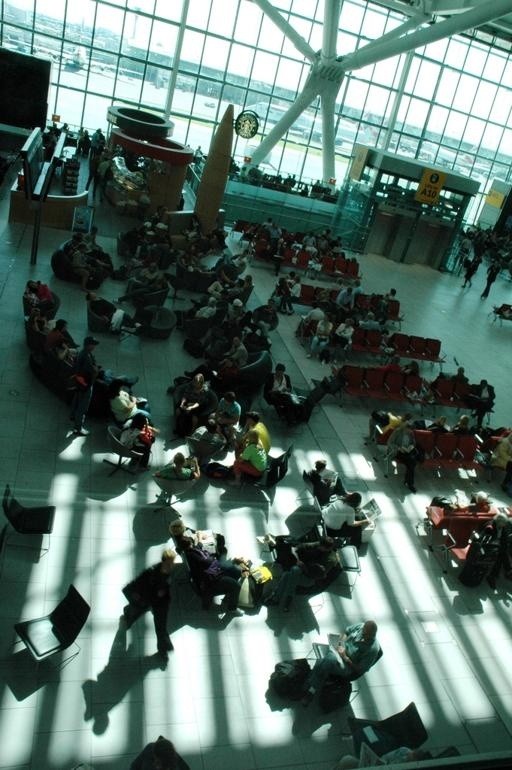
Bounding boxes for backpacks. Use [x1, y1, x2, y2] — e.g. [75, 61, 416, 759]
[205, 462, 232, 480]
[237, 574, 257, 607]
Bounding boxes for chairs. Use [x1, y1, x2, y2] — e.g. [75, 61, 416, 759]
[338, 702, 429, 762]
[7, 583, 91, 676]
[2, 484, 56, 562]
[305, 630, 383, 698]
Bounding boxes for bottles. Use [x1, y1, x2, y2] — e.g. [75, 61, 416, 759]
[190, 456, 196, 472]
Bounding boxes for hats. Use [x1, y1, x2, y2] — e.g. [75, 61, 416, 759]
[83, 336, 101, 347]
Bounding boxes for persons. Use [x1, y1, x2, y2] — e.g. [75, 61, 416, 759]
[1, 121, 511, 769]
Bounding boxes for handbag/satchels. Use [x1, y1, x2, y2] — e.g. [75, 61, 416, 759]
[267, 658, 311, 706]
[122, 582, 151, 612]
[183, 338, 205, 359]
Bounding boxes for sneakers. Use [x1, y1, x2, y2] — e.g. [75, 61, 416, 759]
[402, 480, 418, 493]
[304, 353, 351, 369]
[309, 686, 317, 696]
[70, 414, 90, 436]
[487, 571, 512, 589]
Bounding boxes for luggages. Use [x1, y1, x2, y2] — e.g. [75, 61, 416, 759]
[456, 533, 496, 588]
